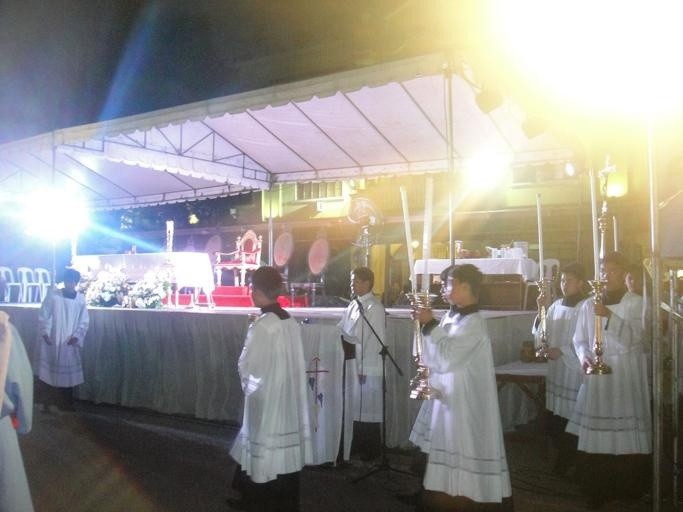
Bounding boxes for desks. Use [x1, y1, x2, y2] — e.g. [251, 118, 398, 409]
[409, 256, 539, 310]
[494, 359, 569, 467]
[71, 252, 217, 308]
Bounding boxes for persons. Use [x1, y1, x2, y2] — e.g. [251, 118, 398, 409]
[410, 260, 514, 511]
[32, 267, 90, 409]
[0, 310, 37, 511]
[407, 262, 463, 500]
[530, 250, 681, 504]
[333, 262, 388, 469]
[224, 264, 312, 512]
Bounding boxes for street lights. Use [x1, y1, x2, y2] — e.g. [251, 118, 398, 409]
[561, 153, 601, 278]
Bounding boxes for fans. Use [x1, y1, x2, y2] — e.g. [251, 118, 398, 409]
[338, 197, 385, 267]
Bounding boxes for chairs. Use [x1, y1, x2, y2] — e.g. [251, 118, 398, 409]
[523, 258, 561, 310]
[0, 267, 52, 303]
[214, 230, 331, 307]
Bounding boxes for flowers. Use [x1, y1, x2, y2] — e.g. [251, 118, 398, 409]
[79, 263, 169, 310]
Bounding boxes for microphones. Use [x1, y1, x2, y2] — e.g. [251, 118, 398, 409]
[350, 293, 363, 312]
